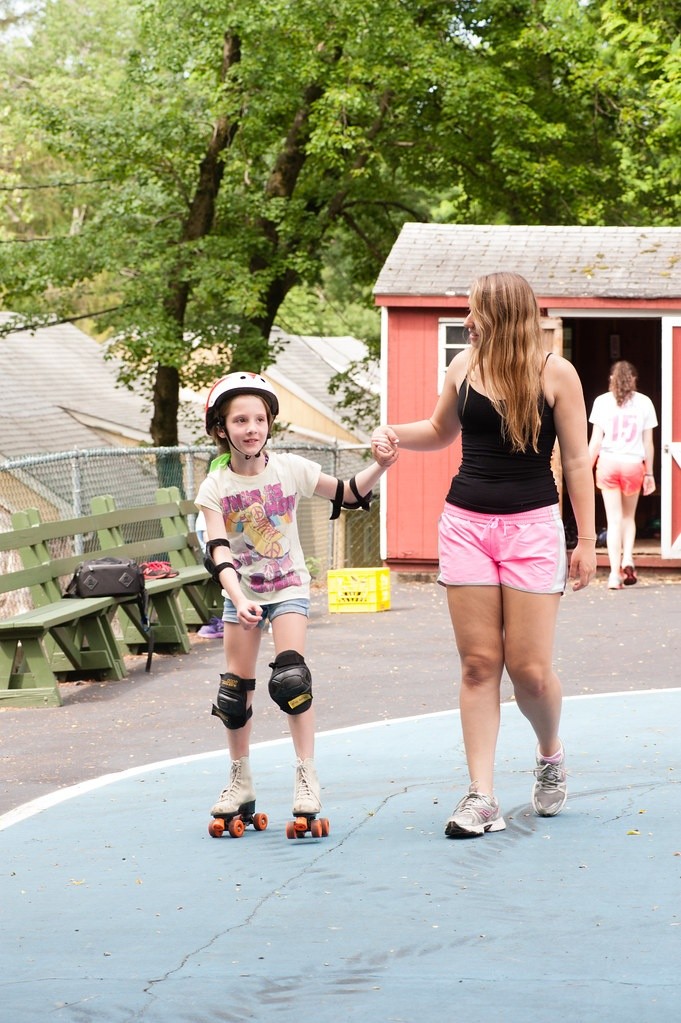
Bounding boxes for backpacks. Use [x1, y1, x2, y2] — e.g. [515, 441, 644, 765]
[64, 556, 149, 631]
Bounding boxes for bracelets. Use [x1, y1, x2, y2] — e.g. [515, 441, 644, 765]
[578, 537, 596, 541]
[645, 474, 653, 476]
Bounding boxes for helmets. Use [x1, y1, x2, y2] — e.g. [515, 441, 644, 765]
[205, 371, 279, 435]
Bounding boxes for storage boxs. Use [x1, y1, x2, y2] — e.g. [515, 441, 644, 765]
[326, 566, 391, 613]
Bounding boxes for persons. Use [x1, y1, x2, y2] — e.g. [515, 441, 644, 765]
[371, 271, 597, 837]
[193, 372, 398, 839]
[588, 361, 659, 590]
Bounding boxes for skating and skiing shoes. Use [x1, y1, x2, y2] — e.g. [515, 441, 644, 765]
[286, 757, 329, 838]
[209, 756, 268, 838]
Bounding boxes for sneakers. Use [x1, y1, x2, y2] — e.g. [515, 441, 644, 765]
[197, 616, 225, 638]
[532, 739, 572, 817]
[444, 780, 506, 834]
[152, 560, 179, 578]
[139, 563, 166, 579]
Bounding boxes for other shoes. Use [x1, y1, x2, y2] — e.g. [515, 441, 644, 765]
[608, 573, 621, 590]
[622, 563, 636, 585]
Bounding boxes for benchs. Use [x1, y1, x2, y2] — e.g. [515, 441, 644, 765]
[0, 486, 215, 709]
